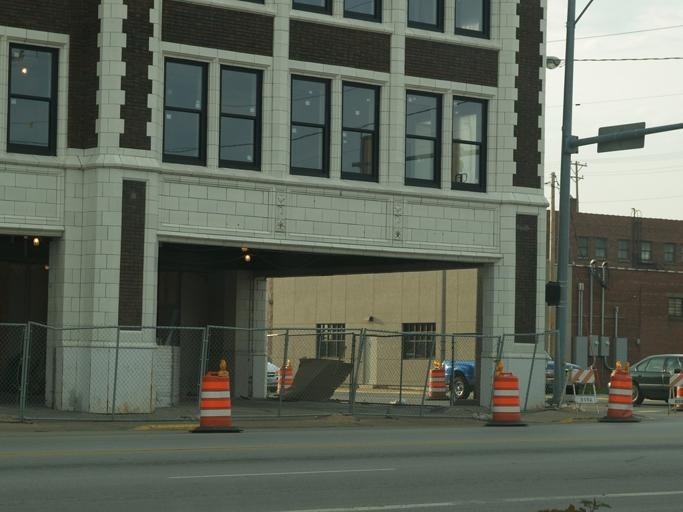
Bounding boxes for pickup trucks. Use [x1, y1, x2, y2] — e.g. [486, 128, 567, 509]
[442, 349, 579, 400]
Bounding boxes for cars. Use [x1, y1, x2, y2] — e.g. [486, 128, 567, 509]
[267, 362, 279, 393]
[607, 354, 683, 405]
